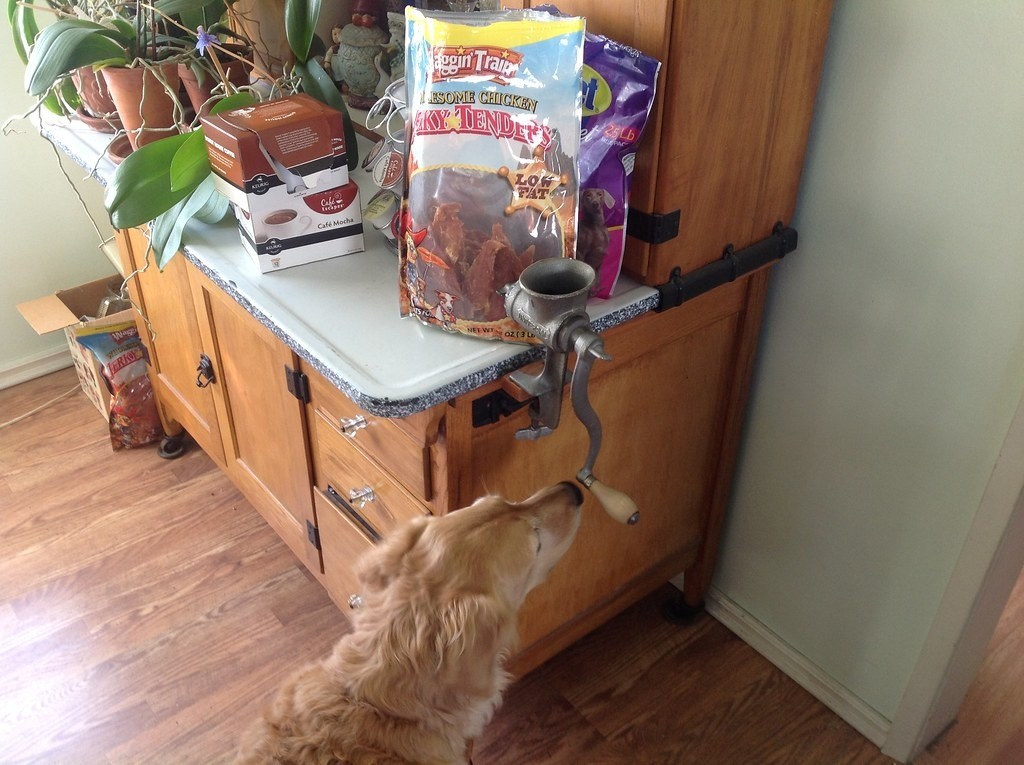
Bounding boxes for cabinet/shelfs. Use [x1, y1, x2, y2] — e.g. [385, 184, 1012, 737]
[48, 119, 800, 692]
[502, 0, 832, 287]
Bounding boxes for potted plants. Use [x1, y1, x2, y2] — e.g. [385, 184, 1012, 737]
[178, 0, 254, 123]
[29, 0, 194, 164]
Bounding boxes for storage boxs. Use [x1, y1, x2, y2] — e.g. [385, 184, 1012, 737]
[16, 273, 141, 423]
[200, 92, 349, 213]
[233, 177, 365, 273]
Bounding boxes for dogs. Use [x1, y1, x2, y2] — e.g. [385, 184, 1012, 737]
[237, 480, 584, 765]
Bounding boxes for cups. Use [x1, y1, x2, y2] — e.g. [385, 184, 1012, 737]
[363, 193, 401, 245]
[475, 0, 499, 11]
[447, 0, 477, 13]
[385, 78, 408, 120]
[373, 151, 402, 198]
[361, 140, 389, 171]
[239, 207, 250, 227]
[260, 209, 312, 239]
